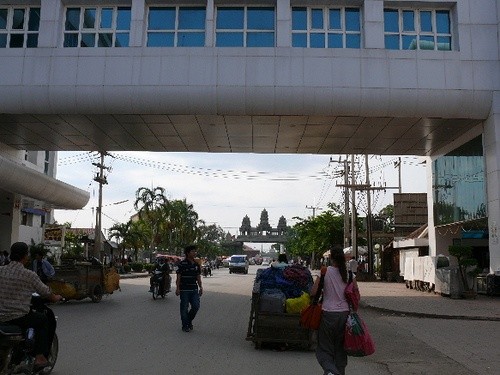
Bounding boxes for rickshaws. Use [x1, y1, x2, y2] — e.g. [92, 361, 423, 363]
[246, 267, 319, 350]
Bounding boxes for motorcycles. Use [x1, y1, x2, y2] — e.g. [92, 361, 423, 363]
[200, 265, 214, 278]
[0, 300, 60, 374]
[151, 272, 172, 303]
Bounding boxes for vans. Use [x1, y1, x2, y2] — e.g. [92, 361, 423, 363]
[228, 255, 250, 274]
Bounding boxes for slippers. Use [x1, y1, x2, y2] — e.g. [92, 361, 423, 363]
[35, 360, 51, 367]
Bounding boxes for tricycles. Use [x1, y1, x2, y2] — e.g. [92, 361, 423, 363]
[39, 263, 122, 304]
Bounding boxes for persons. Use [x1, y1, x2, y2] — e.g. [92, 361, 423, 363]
[149, 256, 172, 293]
[311, 245, 364, 375]
[0, 242, 65, 375]
[201, 259, 211, 276]
[175, 245, 203, 332]
[278, 254, 288, 264]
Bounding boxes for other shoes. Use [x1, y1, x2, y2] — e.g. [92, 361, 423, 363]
[182, 327, 189, 332]
[188, 324, 193, 330]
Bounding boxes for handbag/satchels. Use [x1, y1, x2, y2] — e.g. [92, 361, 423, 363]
[344, 312, 375, 357]
[298, 303, 327, 330]
[344, 271, 360, 311]
